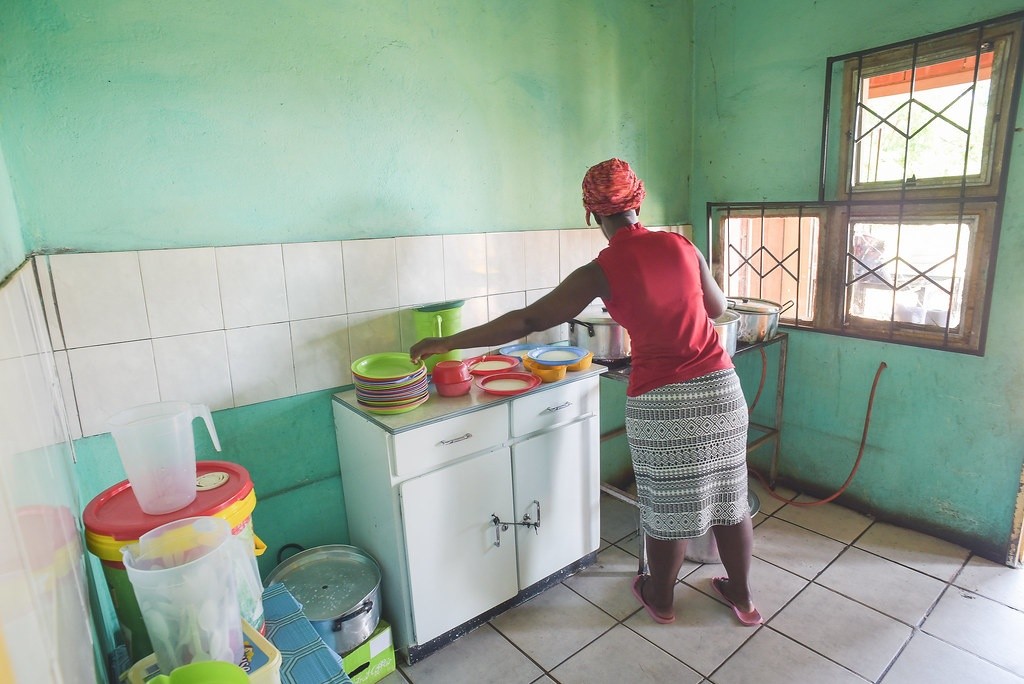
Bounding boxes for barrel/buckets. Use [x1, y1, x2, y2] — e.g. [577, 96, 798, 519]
[82, 461, 265, 660]
[413, 300, 464, 371]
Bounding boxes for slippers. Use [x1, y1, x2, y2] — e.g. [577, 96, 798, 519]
[713, 576, 763, 626]
[631, 574, 675, 624]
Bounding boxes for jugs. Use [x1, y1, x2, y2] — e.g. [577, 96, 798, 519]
[108, 403, 222, 516]
[119, 518, 263, 676]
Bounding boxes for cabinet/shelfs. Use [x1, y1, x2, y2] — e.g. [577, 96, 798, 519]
[330, 362, 608, 664]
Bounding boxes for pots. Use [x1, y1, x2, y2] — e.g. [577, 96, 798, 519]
[685, 490, 761, 563]
[261, 543, 382, 655]
[708, 309, 739, 358]
[566, 305, 634, 368]
[726, 298, 794, 344]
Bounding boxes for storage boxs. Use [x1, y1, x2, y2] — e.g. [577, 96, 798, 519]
[126, 615, 283, 684]
[341, 620, 397, 684]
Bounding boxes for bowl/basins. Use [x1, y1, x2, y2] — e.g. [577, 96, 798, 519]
[431, 361, 471, 384]
[522, 353, 596, 381]
[436, 374, 474, 396]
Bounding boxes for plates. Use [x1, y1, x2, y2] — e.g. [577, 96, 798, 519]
[498, 345, 550, 359]
[350, 352, 430, 415]
[463, 356, 520, 375]
[476, 372, 543, 395]
[527, 346, 589, 365]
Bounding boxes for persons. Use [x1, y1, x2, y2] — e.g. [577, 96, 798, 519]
[410, 157, 762, 626]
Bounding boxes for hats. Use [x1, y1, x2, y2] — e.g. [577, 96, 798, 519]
[583, 158, 645, 226]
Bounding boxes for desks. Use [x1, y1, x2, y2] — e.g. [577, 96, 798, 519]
[108, 582, 354, 684]
[601, 331, 791, 575]
